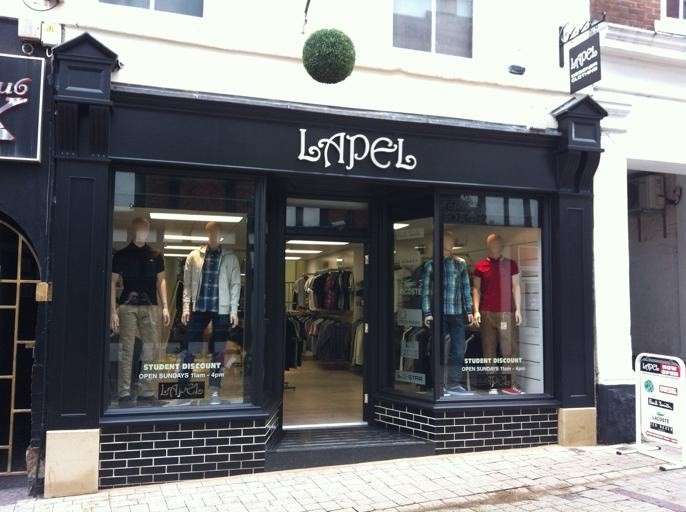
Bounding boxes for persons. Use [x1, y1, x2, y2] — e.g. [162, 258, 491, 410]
[421, 229, 477, 397]
[165, 219, 241, 407]
[470, 233, 529, 395]
[110, 218, 171, 408]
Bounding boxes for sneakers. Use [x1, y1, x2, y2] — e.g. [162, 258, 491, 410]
[487, 387, 499, 395]
[444, 384, 476, 397]
[118, 395, 136, 409]
[207, 391, 222, 405]
[501, 384, 527, 396]
[136, 394, 169, 407]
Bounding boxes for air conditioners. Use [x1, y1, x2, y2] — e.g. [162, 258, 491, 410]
[627, 173, 667, 214]
